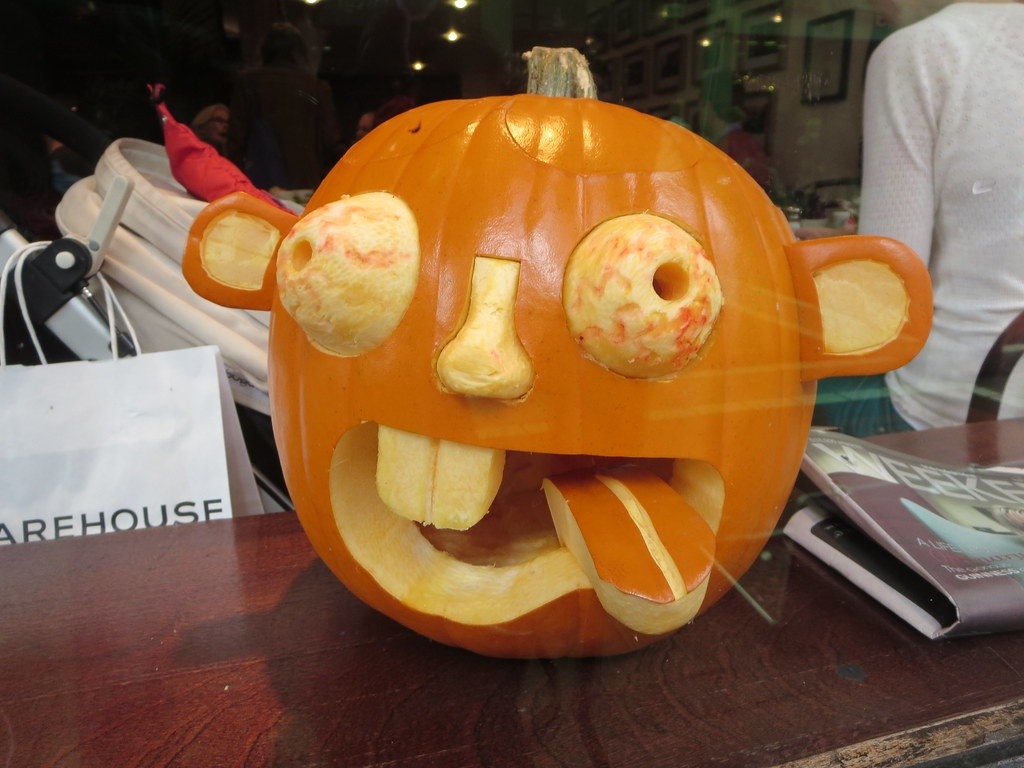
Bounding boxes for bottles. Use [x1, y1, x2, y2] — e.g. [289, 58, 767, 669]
[786, 205, 804, 242]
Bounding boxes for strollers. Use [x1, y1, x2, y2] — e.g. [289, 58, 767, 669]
[0, 138, 305, 513]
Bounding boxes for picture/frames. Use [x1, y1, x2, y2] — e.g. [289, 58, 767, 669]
[799, 9, 854, 105]
[584, 0, 789, 163]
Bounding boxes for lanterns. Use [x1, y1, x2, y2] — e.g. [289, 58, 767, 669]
[180, 46, 934, 660]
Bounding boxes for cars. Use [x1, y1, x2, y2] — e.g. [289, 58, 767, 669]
[773, 179, 861, 231]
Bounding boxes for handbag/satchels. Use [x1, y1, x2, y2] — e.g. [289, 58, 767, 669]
[0, 241, 266, 547]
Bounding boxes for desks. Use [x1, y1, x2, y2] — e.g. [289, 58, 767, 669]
[0, 414, 1024, 768]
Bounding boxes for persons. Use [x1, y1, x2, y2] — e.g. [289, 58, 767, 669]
[698, 68, 776, 195]
[792, 0, 1024, 438]
[190, 103, 282, 194]
[232, 23, 348, 190]
[355, 94, 421, 142]
